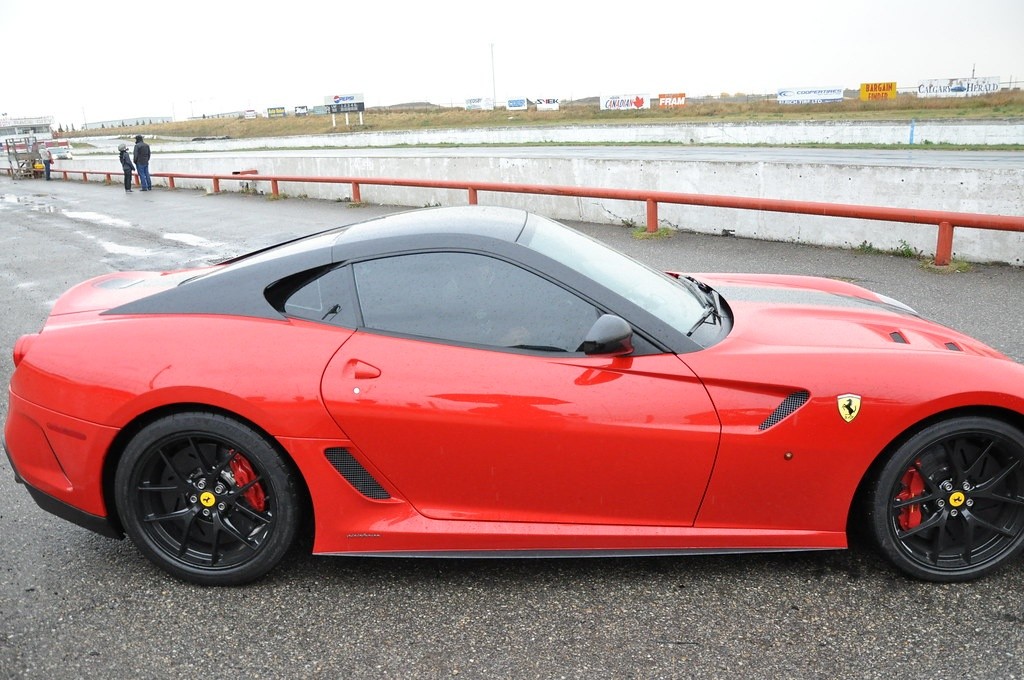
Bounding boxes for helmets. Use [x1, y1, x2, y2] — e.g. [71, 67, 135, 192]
[118, 144, 126, 151]
[135, 135, 143, 144]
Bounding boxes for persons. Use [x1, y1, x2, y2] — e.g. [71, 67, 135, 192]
[133, 135, 151, 191]
[40, 143, 54, 180]
[118, 143, 136, 193]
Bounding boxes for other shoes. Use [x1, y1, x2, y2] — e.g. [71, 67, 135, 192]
[126, 190, 133, 192]
[140, 189, 147, 191]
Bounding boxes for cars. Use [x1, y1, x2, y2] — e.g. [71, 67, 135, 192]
[4, 204, 1024, 583]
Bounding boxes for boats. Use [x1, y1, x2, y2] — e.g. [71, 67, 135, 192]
[0, 114, 72, 159]
